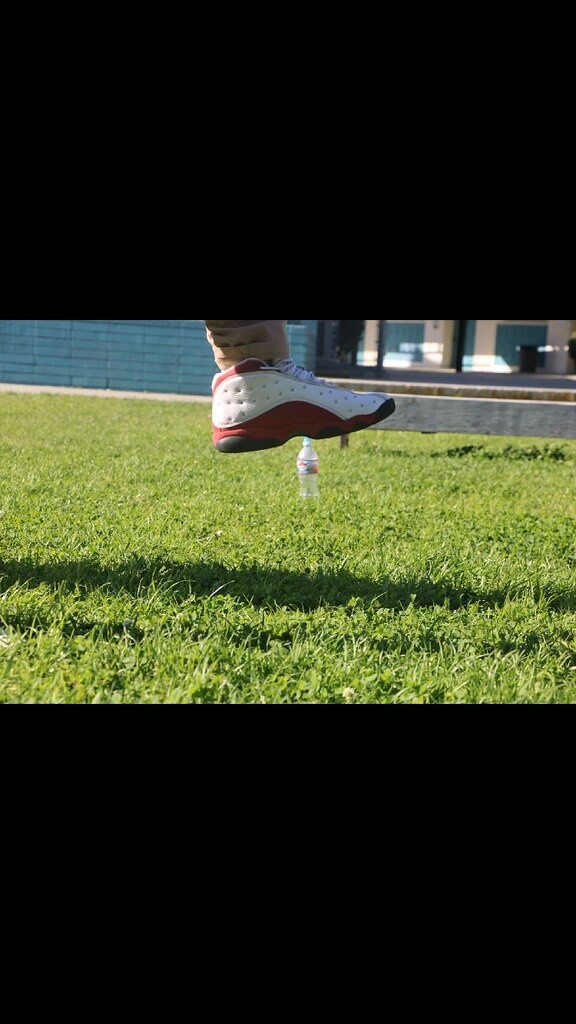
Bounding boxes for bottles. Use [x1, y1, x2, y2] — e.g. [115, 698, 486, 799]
[296, 436, 320, 497]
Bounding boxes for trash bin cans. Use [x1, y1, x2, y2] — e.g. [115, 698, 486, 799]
[519, 344, 539, 373]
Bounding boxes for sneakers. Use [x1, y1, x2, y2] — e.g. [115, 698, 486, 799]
[208, 355, 397, 454]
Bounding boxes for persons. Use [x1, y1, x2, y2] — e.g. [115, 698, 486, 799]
[206, 320, 395, 453]
